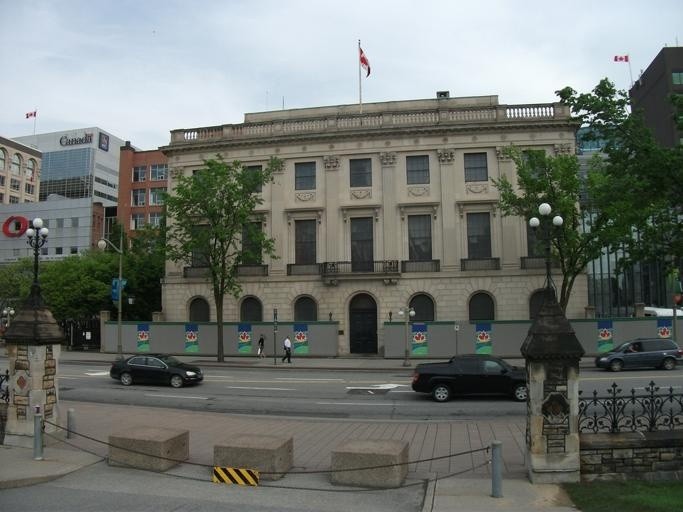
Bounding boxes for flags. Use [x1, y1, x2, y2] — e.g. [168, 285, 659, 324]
[24, 111, 38, 119]
[613, 54, 627, 63]
[359, 48, 370, 78]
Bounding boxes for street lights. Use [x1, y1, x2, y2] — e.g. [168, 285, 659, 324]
[398, 304, 416, 368]
[96, 226, 126, 362]
[526, 201, 564, 294]
[2, 307, 15, 329]
[24, 216, 50, 309]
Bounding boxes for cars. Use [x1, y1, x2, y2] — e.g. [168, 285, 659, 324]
[593, 337, 682, 373]
[410, 355, 529, 403]
[108, 351, 205, 389]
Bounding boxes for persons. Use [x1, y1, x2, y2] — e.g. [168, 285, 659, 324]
[280, 336, 292, 363]
[256, 333, 265, 359]
[629, 342, 641, 351]
